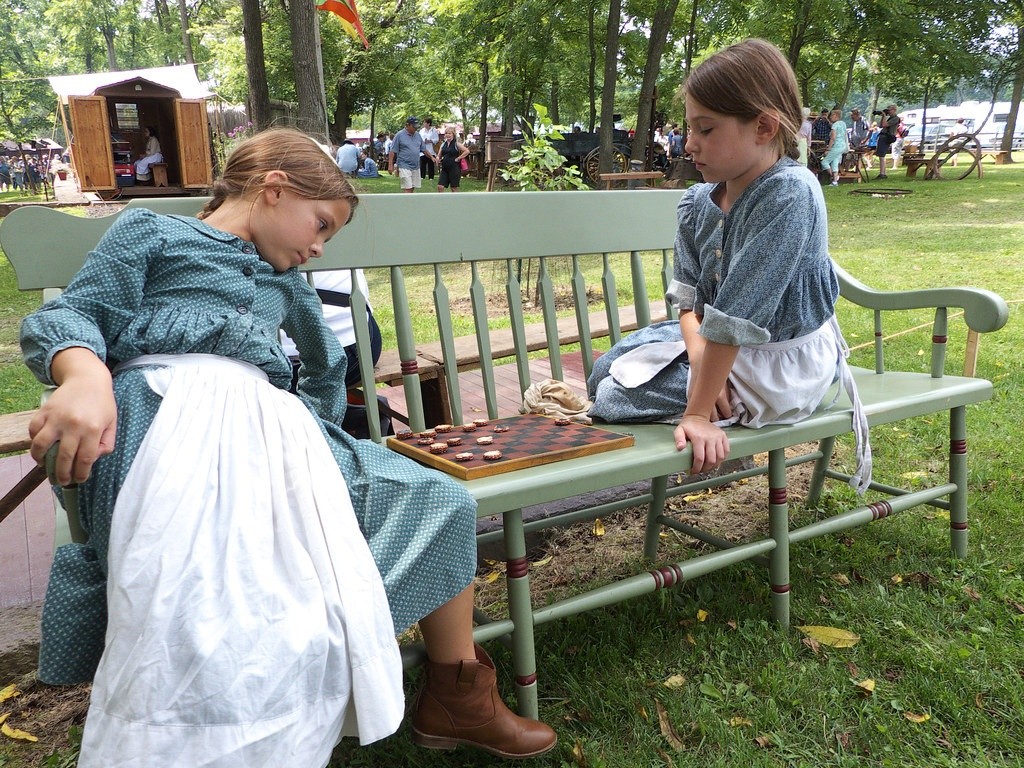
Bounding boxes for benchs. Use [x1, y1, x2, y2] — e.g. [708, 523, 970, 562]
[902, 146, 1024, 178]
[600, 170, 663, 190]
[350, 298, 681, 429]
[0, 190, 1008, 718]
[150, 162, 168, 185]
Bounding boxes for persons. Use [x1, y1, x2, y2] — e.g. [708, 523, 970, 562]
[0, 153, 63, 196]
[20, 127, 557, 768]
[586, 38, 872, 496]
[419, 119, 439, 180]
[435, 126, 470, 192]
[356, 133, 477, 179]
[388, 117, 436, 192]
[133, 125, 164, 185]
[796, 104, 907, 186]
[653, 122, 692, 162]
[948, 118, 967, 167]
[336, 138, 360, 175]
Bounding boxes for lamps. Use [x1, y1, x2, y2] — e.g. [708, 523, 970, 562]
[134, 84, 142, 90]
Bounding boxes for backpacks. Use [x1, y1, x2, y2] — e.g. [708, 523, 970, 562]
[900, 128, 909, 138]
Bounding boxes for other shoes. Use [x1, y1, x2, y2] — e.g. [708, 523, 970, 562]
[872, 174, 887, 180]
[825, 182, 838, 186]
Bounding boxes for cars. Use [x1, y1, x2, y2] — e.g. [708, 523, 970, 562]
[889, 123, 958, 154]
[988, 131, 1024, 151]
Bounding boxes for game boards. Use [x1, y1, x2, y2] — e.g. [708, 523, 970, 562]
[383, 413, 636, 483]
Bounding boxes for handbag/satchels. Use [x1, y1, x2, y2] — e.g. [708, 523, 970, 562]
[14, 163, 23, 172]
[431, 127, 441, 153]
[460, 144, 468, 171]
[843, 134, 850, 154]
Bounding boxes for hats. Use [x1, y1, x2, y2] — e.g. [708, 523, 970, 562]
[42, 155, 47, 158]
[25, 154, 31, 158]
[407, 116, 419, 128]
[54, 154, 60, 158]
[12, 156, 18, 160]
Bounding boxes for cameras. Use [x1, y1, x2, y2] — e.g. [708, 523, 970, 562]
[872, 109, 889, 115]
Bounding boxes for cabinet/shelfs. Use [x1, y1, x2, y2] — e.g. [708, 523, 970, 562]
[113, 141, 130, 165]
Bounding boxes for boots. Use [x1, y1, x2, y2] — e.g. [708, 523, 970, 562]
[412, 641, 558, 757]
